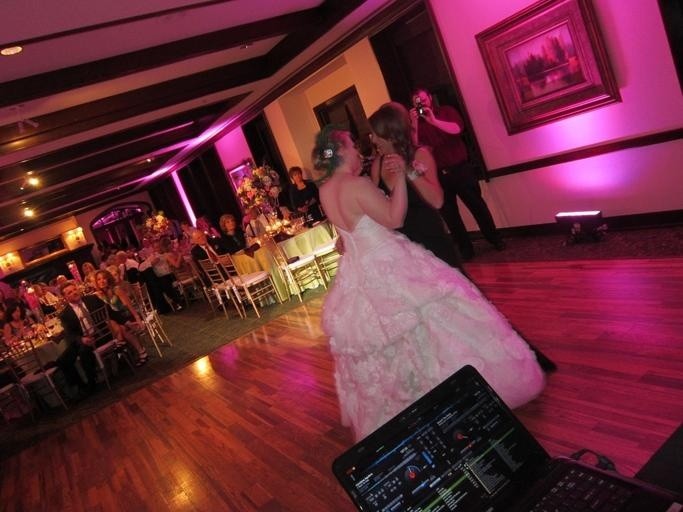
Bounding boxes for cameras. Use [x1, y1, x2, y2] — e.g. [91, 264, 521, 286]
[415, 97, 427, 117]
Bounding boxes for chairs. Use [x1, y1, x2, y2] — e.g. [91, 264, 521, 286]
[196, 258, 250, 320]
[79, 305, 136, 393]
[46, 311, 59, 320]
[0, 384, 30, 419]
[262, 236, 327, 303]
[312, 222, 340, 290]
[216, 253, 282, 319]
[126, 280, 164, 344]
[0, 339, 69, 419]
[178, 270, 204, 310]
[123, 281, 172, 359]
[188, 257, 220, 313]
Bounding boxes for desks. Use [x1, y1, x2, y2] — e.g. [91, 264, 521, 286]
[232, 215, 340, 304]
[0, 316, 69, 409]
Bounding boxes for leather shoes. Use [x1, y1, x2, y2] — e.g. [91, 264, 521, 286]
[489, 240, 503, 250]
[462, 249, 472, 259]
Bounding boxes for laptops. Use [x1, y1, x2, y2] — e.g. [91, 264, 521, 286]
[332, 364, 682, 510]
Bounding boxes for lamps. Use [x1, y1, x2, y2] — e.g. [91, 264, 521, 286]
[553, 210, 608, 247]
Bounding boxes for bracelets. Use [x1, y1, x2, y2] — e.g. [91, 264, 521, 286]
[405, 167, 418, 182]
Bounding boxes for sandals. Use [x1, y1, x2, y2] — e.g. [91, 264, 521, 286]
[135, 350, 151, 367]
[112, 340, 127, 350]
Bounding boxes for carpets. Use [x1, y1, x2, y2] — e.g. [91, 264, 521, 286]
[0, 277, 336, 457]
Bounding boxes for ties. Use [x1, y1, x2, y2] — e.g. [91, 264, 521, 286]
[78, 303, 93, 326]
[205, 246, 218, 263]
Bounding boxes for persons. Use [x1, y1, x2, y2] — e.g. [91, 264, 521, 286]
[195, 215, 220, 238]
[216, 214, 245, 255]
[407, 86, 506, 261]
[179, 221, 195, 240]
[190, 229, 220, 288]
[54, 281, 143, 399]
[94, 270, 151, 368]
[55, 261, 94, 285]
[0, 277, 54, 347]
[146, 284, 187, 316]
[105, 237, 189, 284]
[366, 102, 557, 373]
[287, 166, 319, 217]
[310, 122, 547, 446]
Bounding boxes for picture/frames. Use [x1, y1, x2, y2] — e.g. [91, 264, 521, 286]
[472, 0, 622, 136]
[225, 157, 255, 193]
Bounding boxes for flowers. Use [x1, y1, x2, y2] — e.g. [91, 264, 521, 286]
[144, 210, 169, 240]
[235, 157, 281, 213]
